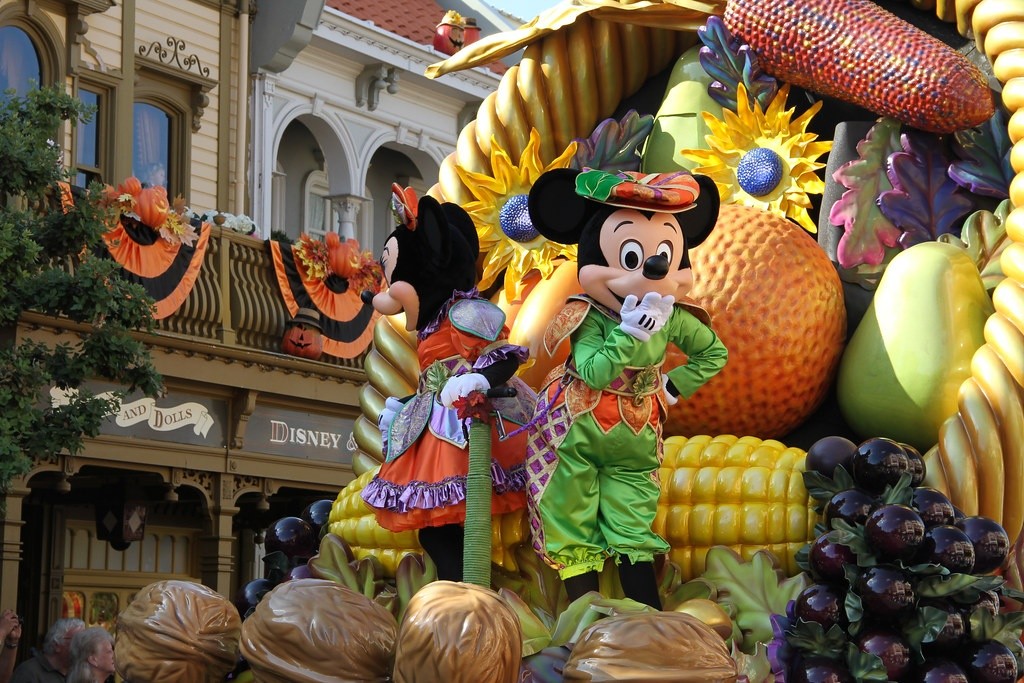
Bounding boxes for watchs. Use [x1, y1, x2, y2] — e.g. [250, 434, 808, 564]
[4, 638, 18, 649]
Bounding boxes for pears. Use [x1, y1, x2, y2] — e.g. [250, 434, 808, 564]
[840, 243, 994, 448]
[641, 47, 722, 177]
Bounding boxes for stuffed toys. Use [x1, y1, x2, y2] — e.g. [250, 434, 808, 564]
[362, 182, 540, 584]
[525, 162, 730, 612]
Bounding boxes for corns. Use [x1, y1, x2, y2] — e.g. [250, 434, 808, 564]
[723, 0, 995, 133]
[322, 436, 816, 605]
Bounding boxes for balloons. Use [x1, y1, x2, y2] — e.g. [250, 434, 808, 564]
[788, 434, 1022, 683]
[240, 497, 340, 609]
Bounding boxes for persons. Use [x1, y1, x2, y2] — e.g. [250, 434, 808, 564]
[65, 627, 116, 683]
[1, 610, 22, 682]
[9, 618, 89, 683]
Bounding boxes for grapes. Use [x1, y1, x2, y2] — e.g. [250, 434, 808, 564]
[776, 434, 1024, 682]
[222, 498, 329, 604]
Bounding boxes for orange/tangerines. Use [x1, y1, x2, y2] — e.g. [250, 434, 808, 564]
[617, 205, 846, 434]
